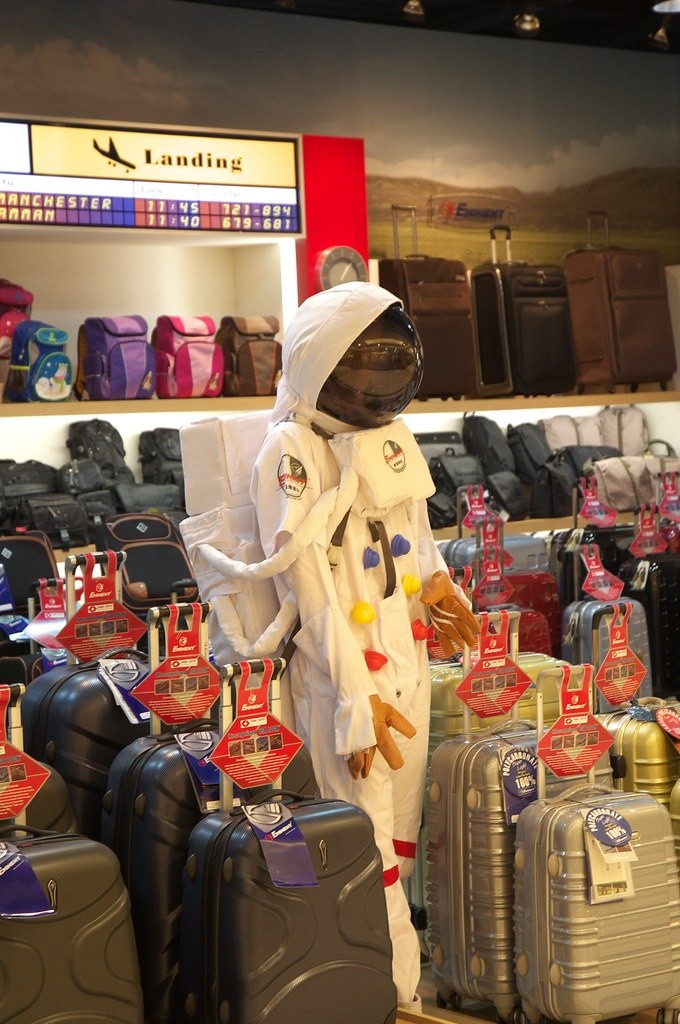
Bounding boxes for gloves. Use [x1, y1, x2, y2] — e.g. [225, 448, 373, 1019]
[420, 570, 481, 656]
[347, 692, 417, 779]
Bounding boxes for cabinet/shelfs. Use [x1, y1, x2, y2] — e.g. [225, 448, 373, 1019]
[1, 395, 278, 562]
[399, 390, 680, 541]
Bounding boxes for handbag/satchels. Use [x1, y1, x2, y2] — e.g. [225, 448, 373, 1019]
[1, 415, 219, 629]
[417, 403, 680, 526]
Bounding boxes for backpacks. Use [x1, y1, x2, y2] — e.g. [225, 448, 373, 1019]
[0, 278, 283, 406]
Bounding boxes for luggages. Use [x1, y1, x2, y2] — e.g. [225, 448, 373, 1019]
[376, 202, 677, 402]
[2, 470, 680, 1024]
